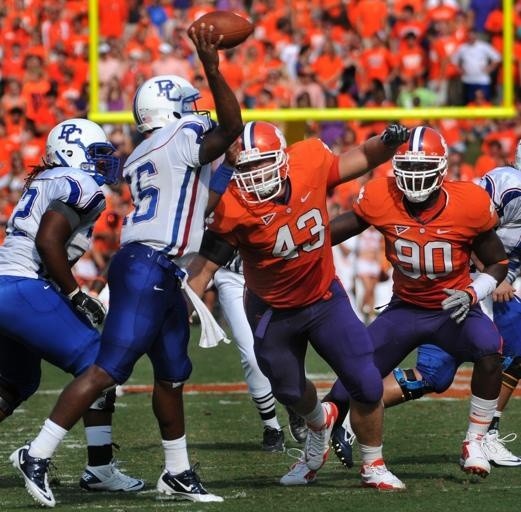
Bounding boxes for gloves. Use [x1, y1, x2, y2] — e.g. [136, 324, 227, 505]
[382, 122, 412, 146]
[69, 287, 106, 327]
[442, 286, 471, 323]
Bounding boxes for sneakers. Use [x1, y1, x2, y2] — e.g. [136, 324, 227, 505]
[262, 424, 285, 450]
[458, 427, 521, 479]
[357, 456, 405, 492]
[7, 444, 58, 510]
[278, 400, 355, 486]
[79, 460, 144, 494]
[155, 468, 224, 504]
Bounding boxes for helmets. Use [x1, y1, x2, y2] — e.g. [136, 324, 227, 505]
[392, 123, 446, 198]
[45, 117, 121, 184]
[231, 121, 292, 203]
[132, 74, 212, 133]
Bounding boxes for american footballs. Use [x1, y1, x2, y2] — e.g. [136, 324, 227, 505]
[188, 11, 255, 48]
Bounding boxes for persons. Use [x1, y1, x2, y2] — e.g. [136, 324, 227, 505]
[10, 23, 245, 508]
[189, 246, 308, 451]
[279, 127, 510, 486]
[331, 135, 521, 470]
[0, 118, 145, 492]
[186, 122, 412, 491]
[0, 2, 520, 326]
